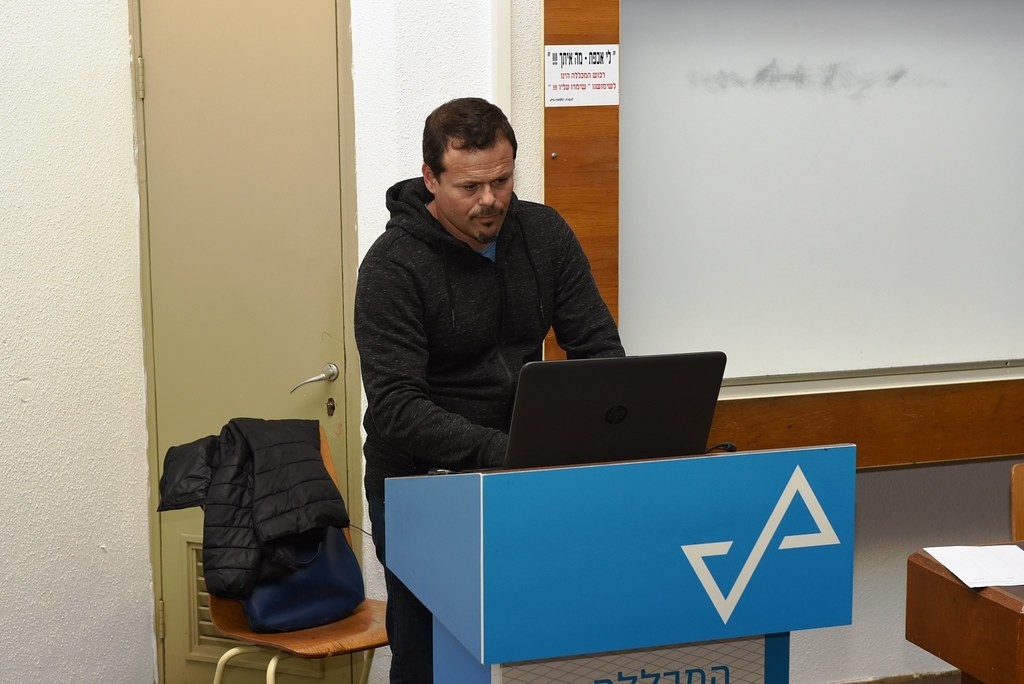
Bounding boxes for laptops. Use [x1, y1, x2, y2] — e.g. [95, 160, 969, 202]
[502, 351, 727, 469]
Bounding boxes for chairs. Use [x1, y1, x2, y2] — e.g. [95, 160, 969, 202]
[209, 424, 390, 684]
[1011, 464, 1024, 541]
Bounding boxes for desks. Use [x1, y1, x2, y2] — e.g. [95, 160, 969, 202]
[905, 540, 1024, 684]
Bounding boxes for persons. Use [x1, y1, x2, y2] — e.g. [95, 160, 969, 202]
[352, 98, 630, 684]
